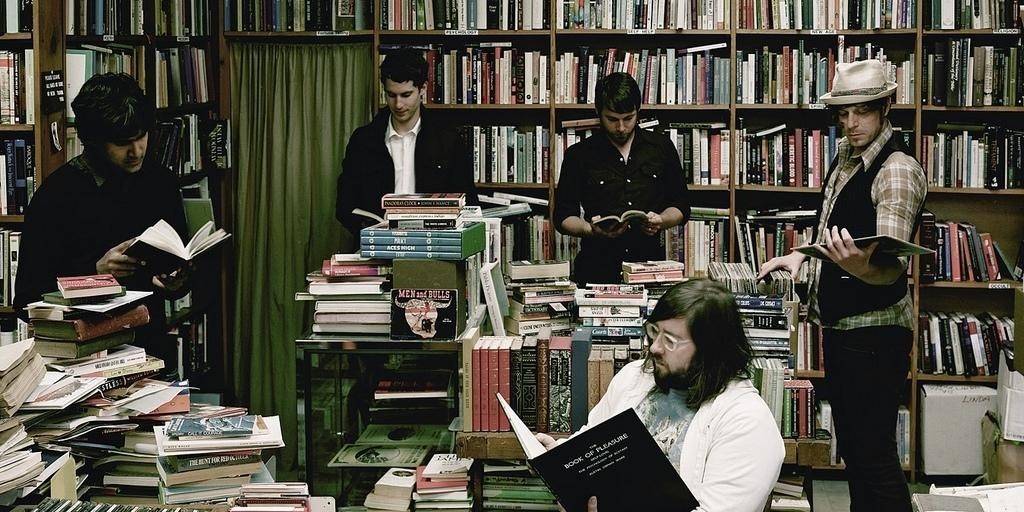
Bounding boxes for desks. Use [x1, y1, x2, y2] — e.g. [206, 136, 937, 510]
[454, 430, 832, 512]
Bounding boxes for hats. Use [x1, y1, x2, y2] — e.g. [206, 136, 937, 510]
[820, 59, 898, 106]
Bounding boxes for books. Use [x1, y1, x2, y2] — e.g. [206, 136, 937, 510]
[296, 46, 1024, 509]
[2, 1, 868, 32]
[0, 35, 307, 512]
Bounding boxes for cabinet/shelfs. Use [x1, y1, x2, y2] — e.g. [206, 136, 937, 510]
[375, 0, 1024, 485]
[295, 331, 463, 509]
[0, 0, 378, 404]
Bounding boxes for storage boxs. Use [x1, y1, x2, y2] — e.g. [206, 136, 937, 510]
[920, 383, 994, 476]
[1011, 282, 1024, 376]
[989, 349, 1024, 449]
[978, 408, 1024, 483]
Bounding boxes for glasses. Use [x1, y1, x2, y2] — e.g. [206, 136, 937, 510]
[645, 322, 692, 352]
